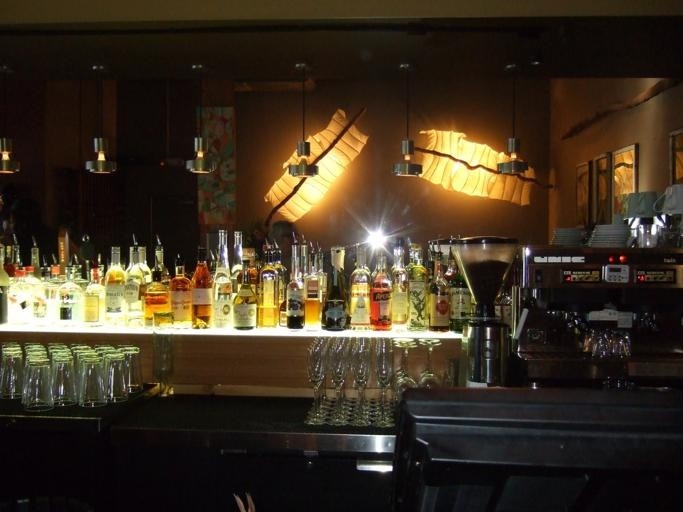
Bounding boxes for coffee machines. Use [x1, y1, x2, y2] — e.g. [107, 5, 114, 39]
[449, 234, 520, 395]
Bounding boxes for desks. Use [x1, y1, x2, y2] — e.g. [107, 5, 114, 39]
[0, 392, 399, 511]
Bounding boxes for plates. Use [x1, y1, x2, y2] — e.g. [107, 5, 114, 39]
[549, 223, 631, 250]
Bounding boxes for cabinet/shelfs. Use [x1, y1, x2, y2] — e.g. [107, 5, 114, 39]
[521, 243, 682, 386]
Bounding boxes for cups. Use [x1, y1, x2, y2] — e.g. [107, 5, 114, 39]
[637, 224, 659, 249]
[0, 339, 141, 411]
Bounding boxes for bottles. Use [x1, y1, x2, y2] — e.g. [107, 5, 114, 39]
[0, 227, 472, 332]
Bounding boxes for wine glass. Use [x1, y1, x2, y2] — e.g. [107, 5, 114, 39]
[297, 332, 395, 429]
[393, 334, 443, 396]
[150, 335, 174, 396]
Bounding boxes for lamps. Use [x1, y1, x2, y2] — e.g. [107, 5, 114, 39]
[621, 181, 683, 247]
